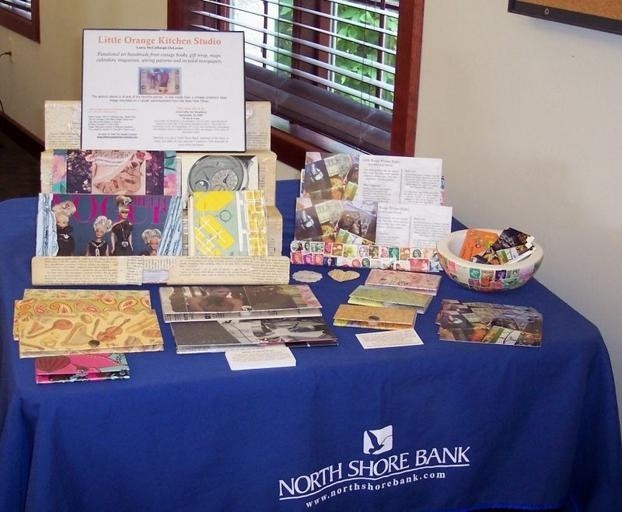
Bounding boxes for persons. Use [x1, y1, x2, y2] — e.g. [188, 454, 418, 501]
[52, 196, 161, 256]
[189, 285, 242, 312]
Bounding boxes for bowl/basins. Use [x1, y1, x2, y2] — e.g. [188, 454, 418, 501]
[436, 227, 544, 293]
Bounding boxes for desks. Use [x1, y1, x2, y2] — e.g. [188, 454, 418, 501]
[1, 197, 605, 512]
